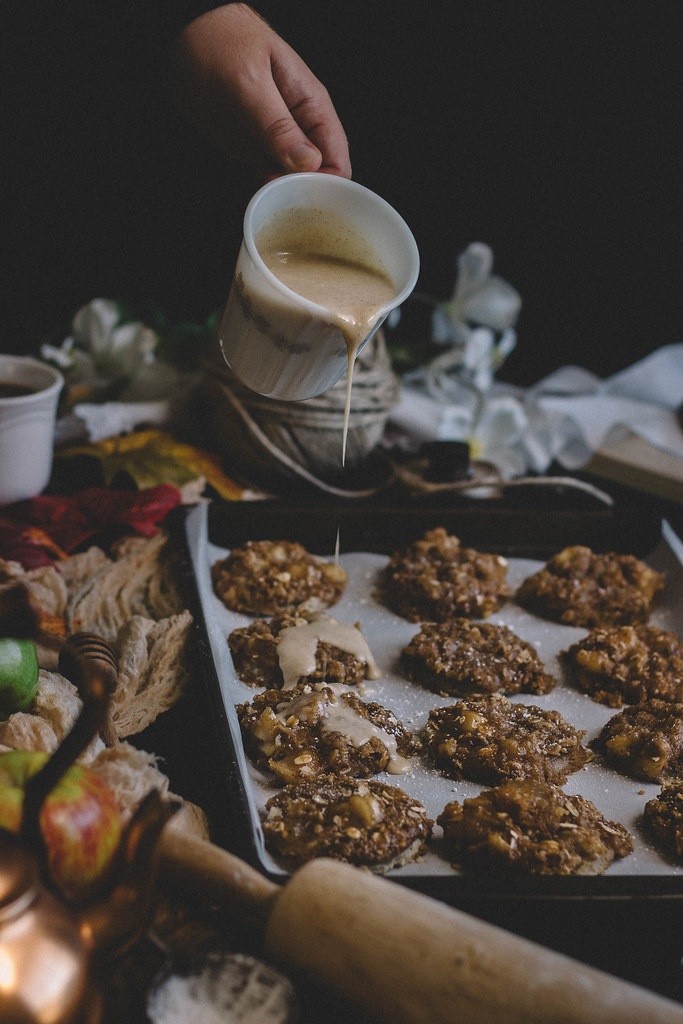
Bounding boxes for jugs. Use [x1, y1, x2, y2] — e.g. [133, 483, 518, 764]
[218, 174, 420, 402]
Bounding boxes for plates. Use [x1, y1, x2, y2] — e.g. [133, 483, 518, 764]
[184, 504, 682, 902]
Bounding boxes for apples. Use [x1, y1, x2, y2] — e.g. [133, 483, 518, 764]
[0, 637, 40, 716]
[0, 751, 119, 891]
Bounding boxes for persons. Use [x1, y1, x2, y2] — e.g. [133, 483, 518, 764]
[153, 0, 351, 184]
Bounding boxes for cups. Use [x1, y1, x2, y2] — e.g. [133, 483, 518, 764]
[0, 354, 65, 503]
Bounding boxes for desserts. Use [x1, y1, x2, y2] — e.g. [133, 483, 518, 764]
[211, 528, 683, 874]
